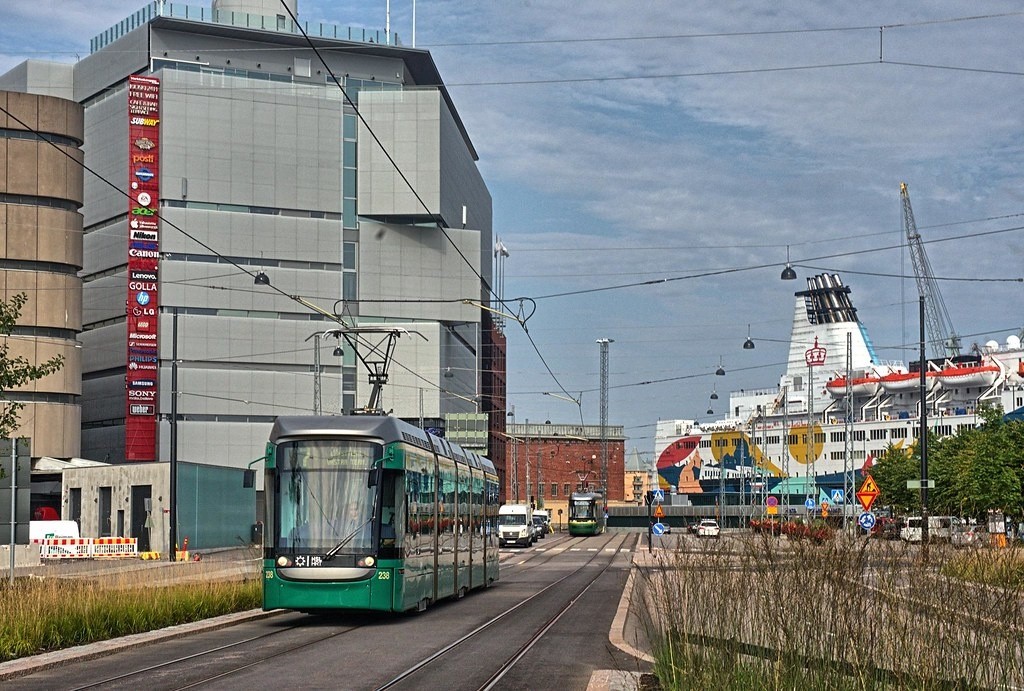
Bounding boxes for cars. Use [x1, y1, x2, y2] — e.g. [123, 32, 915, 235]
[662, 523, 671, 534]
[698, 518, 720, 539]
[532, 517, 546, 538]
[857, 513, 898, 540]
[953, 526, 992, 548]
[686, 521, 699, 533]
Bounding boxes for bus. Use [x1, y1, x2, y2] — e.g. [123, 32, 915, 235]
[568, 492, 605, 536]
[242, 413, 502, 619]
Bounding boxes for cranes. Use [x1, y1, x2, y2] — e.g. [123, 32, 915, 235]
[896, 184, 967, 358]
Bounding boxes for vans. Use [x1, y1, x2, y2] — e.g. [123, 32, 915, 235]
[532, 511, 551, 533]
[900, 516, 965, 543]
[498, 504, 534, 548]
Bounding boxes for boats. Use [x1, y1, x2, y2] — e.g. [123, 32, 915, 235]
[825, 367, 881, 399]
[878, 367, 934, 391]
[935, 356, 1004, 389]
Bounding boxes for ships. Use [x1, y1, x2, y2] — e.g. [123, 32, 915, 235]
[651, 272, 1024, 506]
